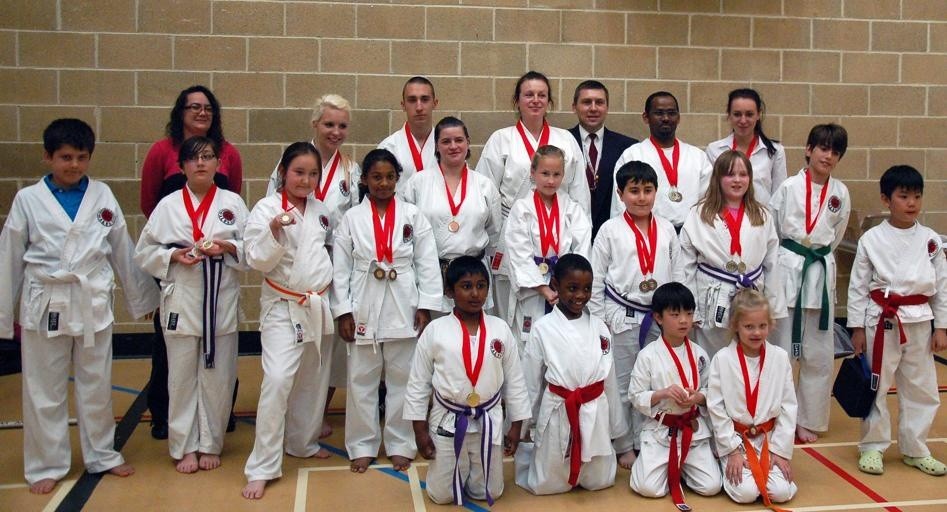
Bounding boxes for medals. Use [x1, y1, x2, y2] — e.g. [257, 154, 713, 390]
[279, 212, 293, 225]
[191, 240, 214, 257]
[800, 236, 812, 249]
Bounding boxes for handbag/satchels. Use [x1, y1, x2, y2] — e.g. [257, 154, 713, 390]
[833, 355, 877, 417]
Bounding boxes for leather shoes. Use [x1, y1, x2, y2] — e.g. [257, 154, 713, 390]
[151, 418, 168, 438]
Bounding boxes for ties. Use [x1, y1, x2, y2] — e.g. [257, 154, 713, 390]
[586, 133, 597, 189]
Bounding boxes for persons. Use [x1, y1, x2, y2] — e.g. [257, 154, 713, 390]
[139, 84, 243, 439]
[265, 94, 363, 439]
[134, 134, 251, 475]
[846, 164, 947, 477]
[333, 69, 798, 506]
[0, 118, 161, 496]
[243, 140, 334, 500]
[766, 122, 852, 445]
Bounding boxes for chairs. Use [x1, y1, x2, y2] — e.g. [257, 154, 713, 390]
[832, 210, 890, 256]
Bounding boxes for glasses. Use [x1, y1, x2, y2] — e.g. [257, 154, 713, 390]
[185, 102, 212, 113]
[185, 153, 215, 161]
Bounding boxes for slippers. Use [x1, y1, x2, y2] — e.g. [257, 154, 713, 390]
[904, 454, 947, 475]
[859, 449, 884, 474]
